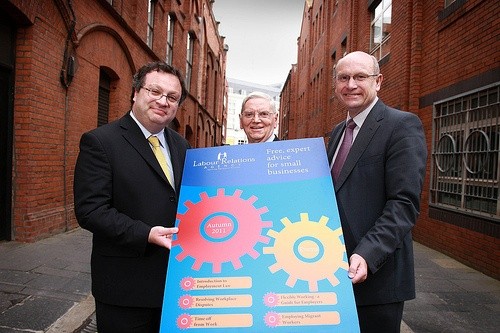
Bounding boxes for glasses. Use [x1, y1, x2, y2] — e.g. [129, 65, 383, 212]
[141, 86, 181, 105]
[242, 111, 275, 118]
[333, 73, 377, 82]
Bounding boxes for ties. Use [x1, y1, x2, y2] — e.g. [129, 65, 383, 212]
[147, 136, 172, 186]
[331, 119, 357, 185]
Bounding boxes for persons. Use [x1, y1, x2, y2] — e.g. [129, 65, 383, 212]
[72, 62, 192, 333]
[238, 92, 281, 144]
[326, 50, 428, 333]
